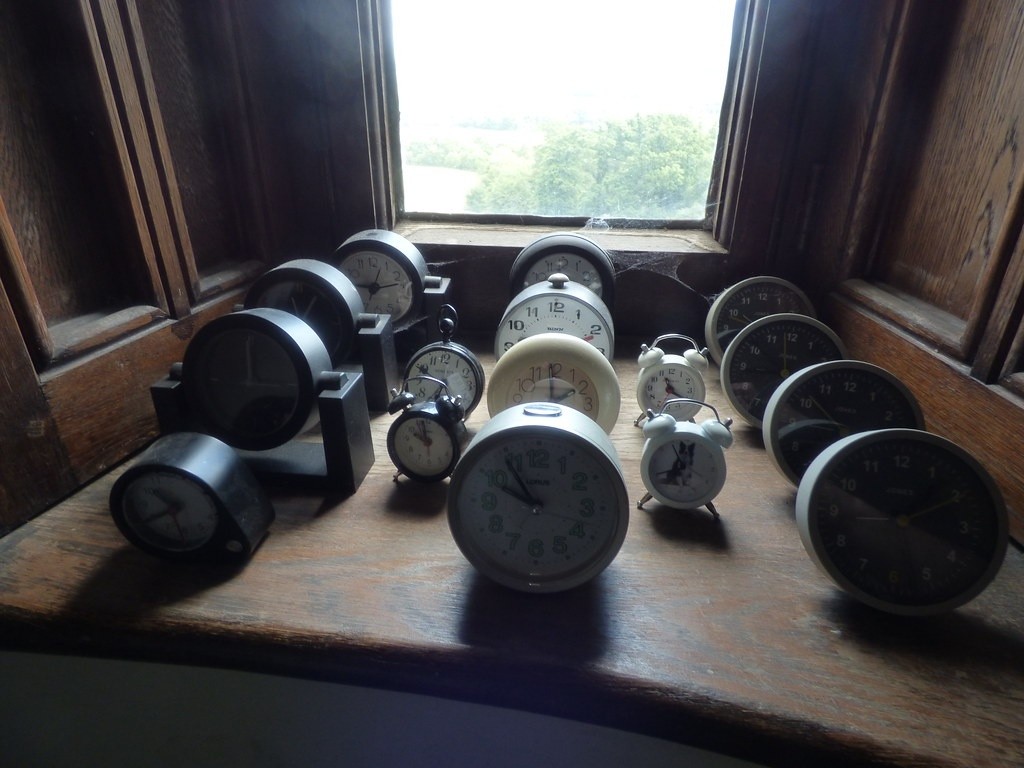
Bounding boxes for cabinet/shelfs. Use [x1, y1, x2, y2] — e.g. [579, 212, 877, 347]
[0, 0, 303, 538]
[793, 0, 1024, 550]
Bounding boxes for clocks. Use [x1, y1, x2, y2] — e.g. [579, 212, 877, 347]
[507, 232, 616, 309]
[633, 331, 709, 426]
[325, 230, 452, 359]
[720, 312, 850, 431]
[794, 426, 1009, 617]
[447, 399, 630, 594]
[231, 258, 399, 411]
[495, 274, 616, 364]
[487, 331, 622, 436]
[149, 307, 373, 493]
[762, 359, 928, 492]
[704, 276, 815, 370]
[402, 303, 484, 436]
[385, 376, 466, 488]
[636, 397, 733, 520]
[110, 432, 274, 565]
